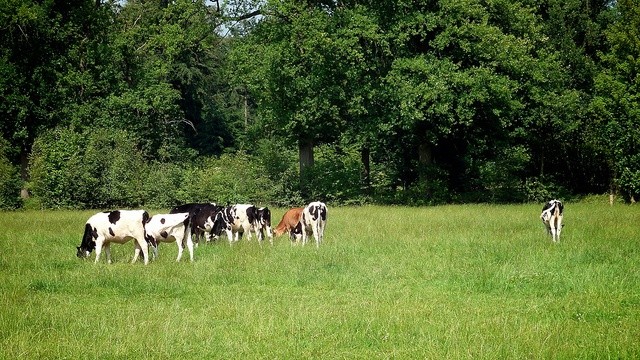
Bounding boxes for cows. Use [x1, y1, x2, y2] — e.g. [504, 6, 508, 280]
[213, 203, 262, 248]
[169, 202, 221, 247]
[139, 212, 194, 264]
[540, 199, 565, 242]
[76, 209, 150, 267]
[289, 201, 328, 249]
[272, 208, 306, 240]
[237, 207, 274, 246]
